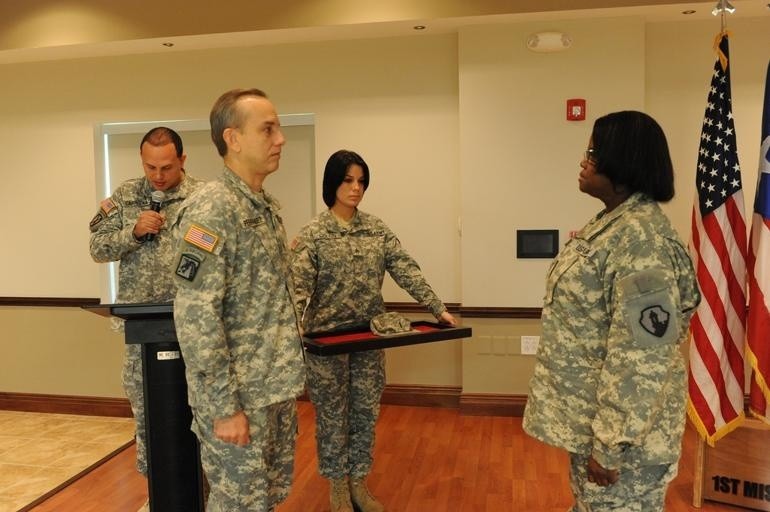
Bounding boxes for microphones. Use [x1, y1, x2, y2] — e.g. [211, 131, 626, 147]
[145, 190, 165, 241]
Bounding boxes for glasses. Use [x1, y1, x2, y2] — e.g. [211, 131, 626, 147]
[583, 148, 603, 163]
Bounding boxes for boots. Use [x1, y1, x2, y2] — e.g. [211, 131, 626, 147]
[137, 498, 150, 512]
[329, 479, 384, 512]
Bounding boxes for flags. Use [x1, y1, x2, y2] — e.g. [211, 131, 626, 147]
[685, 31, 746, 447]
[746, 61, 770, 427]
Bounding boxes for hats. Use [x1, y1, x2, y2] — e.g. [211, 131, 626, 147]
[369, 312, 422, 336]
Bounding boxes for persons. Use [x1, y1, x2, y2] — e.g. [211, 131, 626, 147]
[288, 150, 457, 512]
[88, 126, 208, 512]
[170, 88, 306, 511]
[522, 111, 701, 512]
